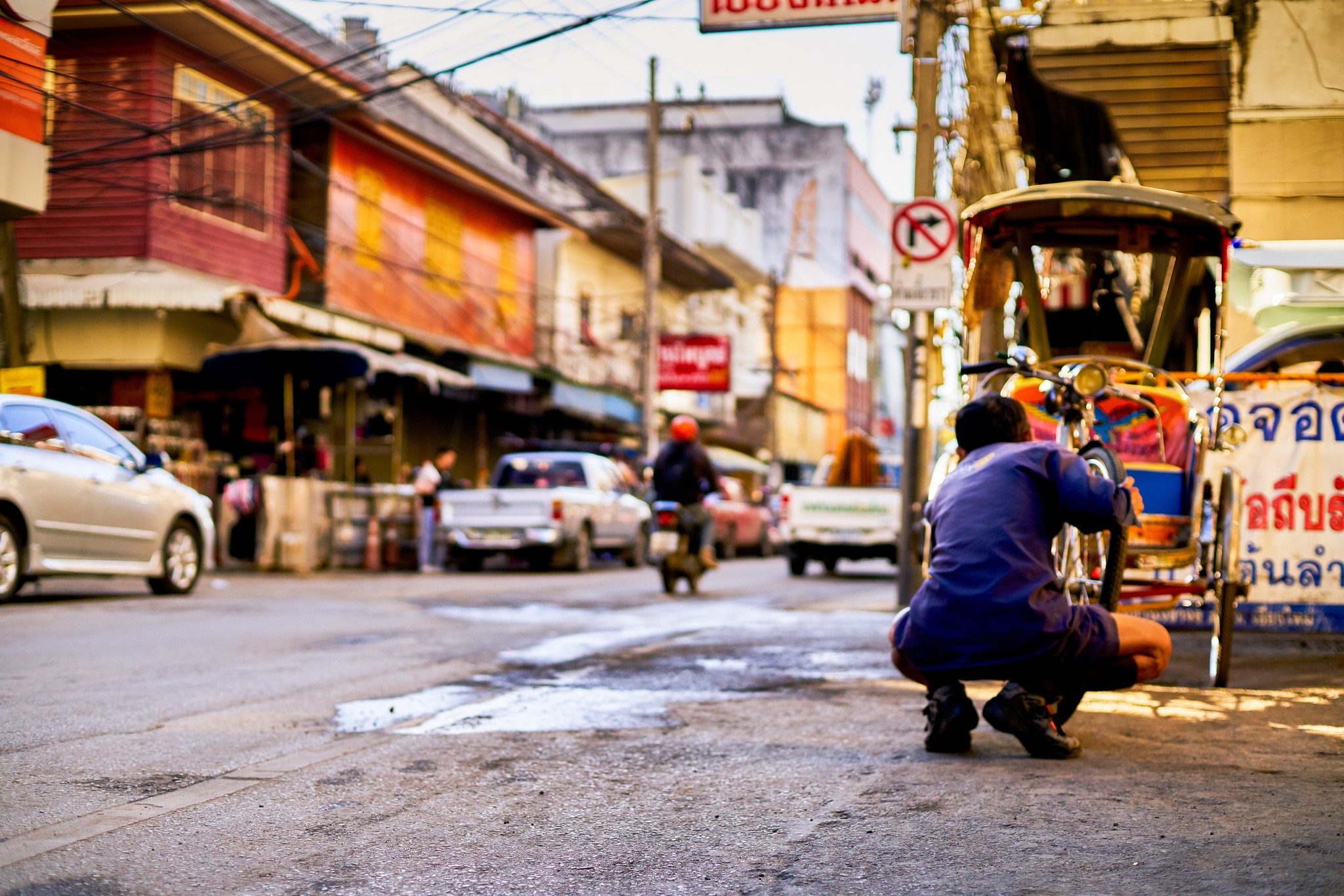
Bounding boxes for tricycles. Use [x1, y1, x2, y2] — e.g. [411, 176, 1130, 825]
[924, 179, 1253, 688]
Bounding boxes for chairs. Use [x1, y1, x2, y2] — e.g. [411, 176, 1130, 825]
[999, 351, 1211, 523]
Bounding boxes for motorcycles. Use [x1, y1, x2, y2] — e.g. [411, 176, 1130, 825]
[647, 496, 707, 595]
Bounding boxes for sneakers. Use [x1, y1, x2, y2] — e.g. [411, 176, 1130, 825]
[922, 682, 979, 754]
[982, 681, 1083, 759]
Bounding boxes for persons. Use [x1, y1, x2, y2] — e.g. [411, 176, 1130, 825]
[276, 418, 472, 575]
[886, 394, 1173, 759]
[651, 414, 730, 569]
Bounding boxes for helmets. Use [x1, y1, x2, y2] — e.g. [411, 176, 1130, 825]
[669, 415, 699, 443]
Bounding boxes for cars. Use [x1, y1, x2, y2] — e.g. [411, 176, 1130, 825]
[1188, 315, 1343, 390]
[0, 394, 216, 606]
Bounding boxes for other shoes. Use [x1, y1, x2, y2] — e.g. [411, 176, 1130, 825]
[699, 547, 718, 568]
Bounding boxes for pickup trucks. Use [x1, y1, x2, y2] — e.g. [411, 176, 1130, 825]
[701, 444, 902, 577]
[432, 450, 653, 573]
